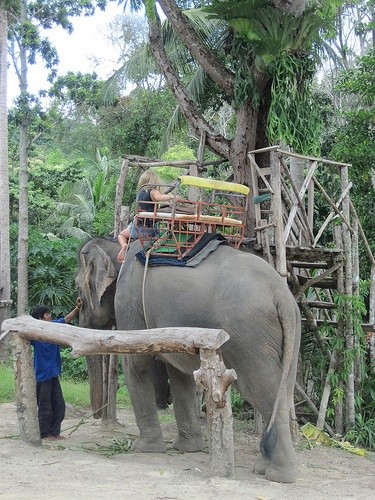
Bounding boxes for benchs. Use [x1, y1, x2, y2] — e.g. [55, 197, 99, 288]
[132, 176, 251, 259]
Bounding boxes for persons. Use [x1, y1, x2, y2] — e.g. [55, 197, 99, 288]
[117, 170, 187, 265]
[17, 295, 85, 441]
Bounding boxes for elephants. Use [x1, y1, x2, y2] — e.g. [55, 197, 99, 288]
[75, 237, 303, 483]
